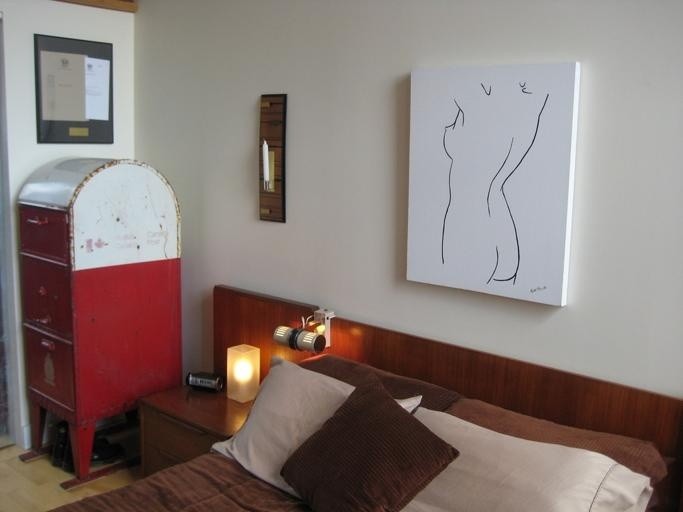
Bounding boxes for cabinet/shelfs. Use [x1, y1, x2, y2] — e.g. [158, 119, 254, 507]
[15, 155, 183, 491]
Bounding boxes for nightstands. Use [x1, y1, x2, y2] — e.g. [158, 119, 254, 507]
[137, 386, 255, 479]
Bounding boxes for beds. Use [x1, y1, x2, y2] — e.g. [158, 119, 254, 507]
[45, 354, 682, 512]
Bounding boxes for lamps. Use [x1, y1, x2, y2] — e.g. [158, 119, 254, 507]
[225, 344, 260, 403]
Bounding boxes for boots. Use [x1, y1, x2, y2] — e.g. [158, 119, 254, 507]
[50, 421, 67, 467]
[61, 432, 125, 472]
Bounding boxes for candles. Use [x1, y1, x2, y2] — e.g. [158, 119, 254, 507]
[262, 137, 268, 183]
